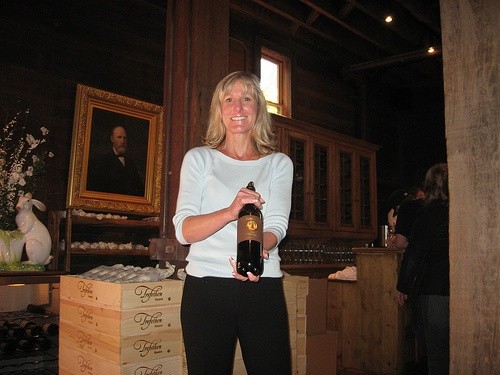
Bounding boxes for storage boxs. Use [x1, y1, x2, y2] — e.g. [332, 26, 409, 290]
[58, 274, 338, 375]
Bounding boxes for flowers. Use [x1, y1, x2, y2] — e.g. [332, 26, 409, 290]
[0, 105, 55, 230]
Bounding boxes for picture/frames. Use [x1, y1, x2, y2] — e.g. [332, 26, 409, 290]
[66, 83, 163, 217]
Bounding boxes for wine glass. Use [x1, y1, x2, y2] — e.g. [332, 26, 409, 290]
[279, 243, 327, 265]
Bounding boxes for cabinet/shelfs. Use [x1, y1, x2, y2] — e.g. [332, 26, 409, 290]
[0, 208, 159, 375]
[270, 113, 383, 279]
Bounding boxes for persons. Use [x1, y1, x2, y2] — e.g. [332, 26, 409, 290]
[172, 70, 295, 375]
[394, 163, 450, 375]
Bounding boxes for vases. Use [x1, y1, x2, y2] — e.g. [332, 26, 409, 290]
[0, 229, 25, 264]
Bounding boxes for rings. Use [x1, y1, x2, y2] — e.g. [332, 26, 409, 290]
[255, 199, 259, 203]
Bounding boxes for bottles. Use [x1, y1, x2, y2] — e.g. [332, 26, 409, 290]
[0, 320, 59, 339]
[236, 185, 263, 277]
[27, 304, 54, 316]
[0, 336, 59, 355]
[387, 229, 397, 248]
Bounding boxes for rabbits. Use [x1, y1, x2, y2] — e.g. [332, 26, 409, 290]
[15, 193, 54, 266]
